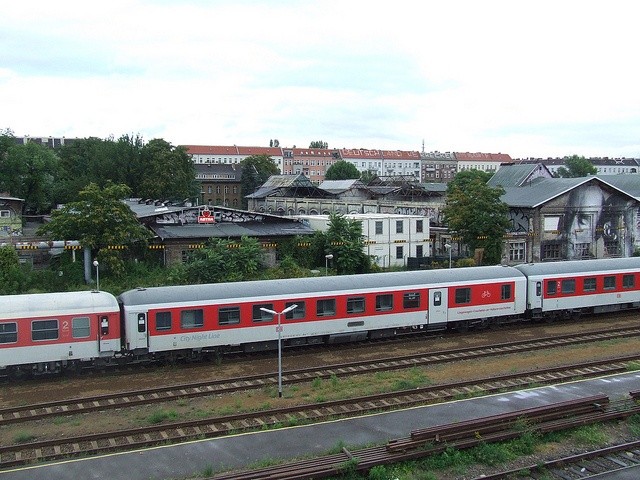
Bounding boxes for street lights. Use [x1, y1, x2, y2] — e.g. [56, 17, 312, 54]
[445, 244, 451, 268]
[259, 303, 299, 398]
[93, 261, 99, 290]
[325, 254, 333, 276]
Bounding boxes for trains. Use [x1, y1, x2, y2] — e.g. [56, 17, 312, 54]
[0, 256, 639, 383]
